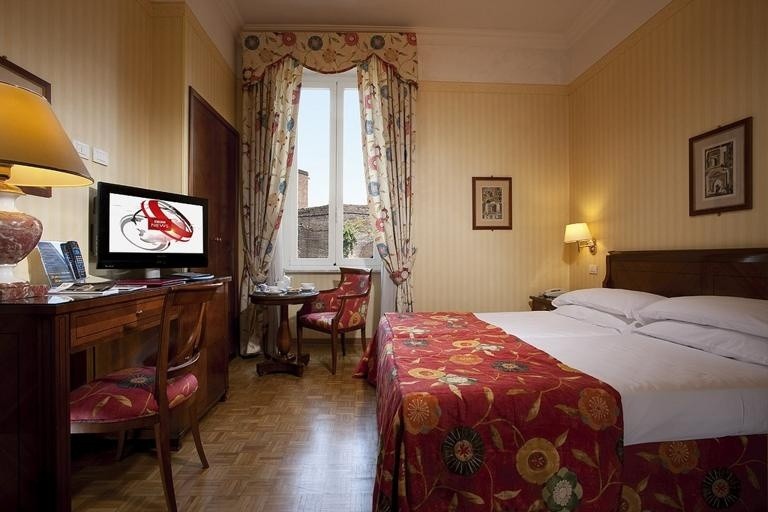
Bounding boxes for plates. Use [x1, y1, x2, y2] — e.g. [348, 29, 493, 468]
[263, 291, 283, 294]
[300, 287, 313, 291]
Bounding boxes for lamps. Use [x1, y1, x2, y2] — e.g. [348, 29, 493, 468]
[0, 82, 94, 300]
[563, 223, 597, 256]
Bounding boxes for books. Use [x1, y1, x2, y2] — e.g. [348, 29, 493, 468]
[47, 281, 115, 294]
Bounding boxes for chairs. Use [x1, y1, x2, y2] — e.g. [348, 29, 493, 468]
[68, 282, 224, 512]
[296, 267, 373, 375]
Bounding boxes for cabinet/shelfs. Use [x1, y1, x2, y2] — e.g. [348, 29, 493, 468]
[0, 275, 231, 512]
[529, 295, 558, 312]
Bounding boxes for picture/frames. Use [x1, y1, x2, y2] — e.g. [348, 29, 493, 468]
[0, 52, 55, 199]
[471, 176, 512, 231]
[689, 117, 753, 217]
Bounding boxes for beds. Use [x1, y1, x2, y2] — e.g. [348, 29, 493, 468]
[380, 249, 768, 511]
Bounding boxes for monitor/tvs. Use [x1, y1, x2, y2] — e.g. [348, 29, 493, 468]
[92, 181, 209, 280]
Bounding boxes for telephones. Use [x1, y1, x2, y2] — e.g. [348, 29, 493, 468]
[68, 238, 86, 279]
[543, 288, 566, 298]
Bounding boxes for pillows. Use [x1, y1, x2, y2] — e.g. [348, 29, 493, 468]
[639, 296, 767, 337]
[633, 320, 767, 366]
[551, 288, 667, 320]
[552, 307, 634, 334]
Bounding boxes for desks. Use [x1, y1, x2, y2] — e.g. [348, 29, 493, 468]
[250, 287, 319, 376]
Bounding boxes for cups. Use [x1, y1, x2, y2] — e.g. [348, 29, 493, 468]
[300, 283, 314, 288]
[269, 286, 279, 292]
